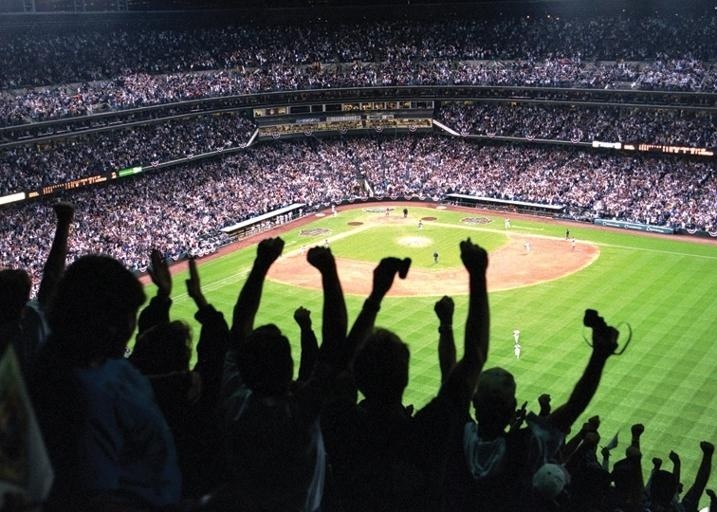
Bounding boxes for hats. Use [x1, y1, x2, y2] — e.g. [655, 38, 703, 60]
[474, 368, 514, 416]
[532, 463, 566, 497]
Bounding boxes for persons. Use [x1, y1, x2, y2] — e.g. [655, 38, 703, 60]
[0, 1, 717, 510]
